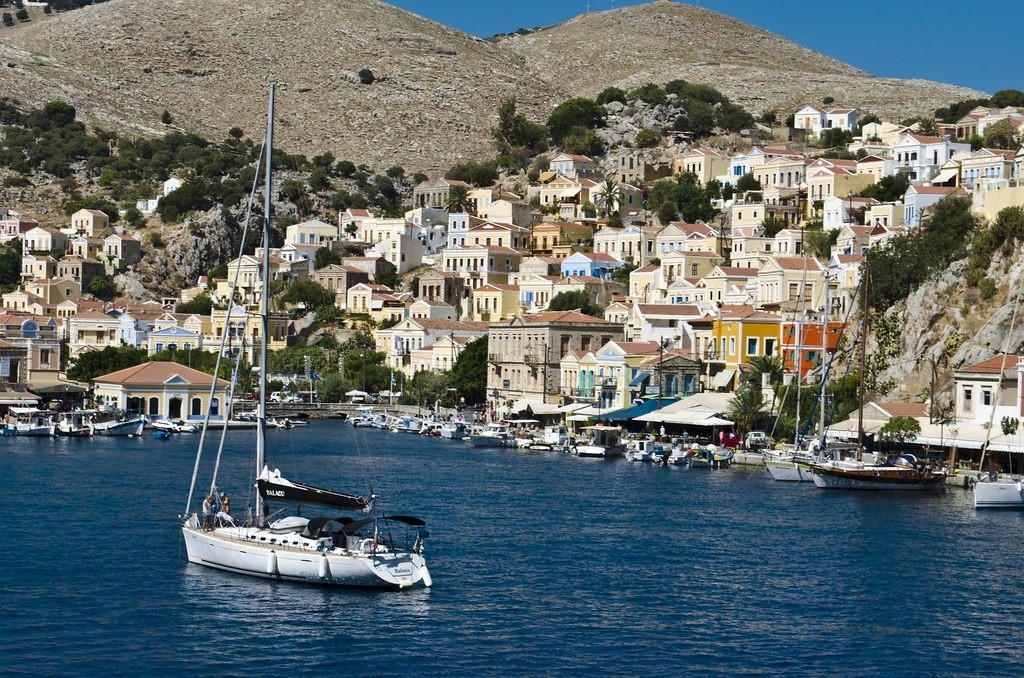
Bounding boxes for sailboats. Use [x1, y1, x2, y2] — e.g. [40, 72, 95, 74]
[763, 252, 947, 488]
[178, 80, 435, 592]
[972, 278, 1024, 508]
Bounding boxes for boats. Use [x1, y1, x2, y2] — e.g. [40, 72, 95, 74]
[0, 407, 146, 435]
[275, 420, 308, 429]
[345, 410, 737, 469]
[151, 421, 199, 432]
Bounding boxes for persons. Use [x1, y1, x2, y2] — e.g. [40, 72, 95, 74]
[473, 407, 495, 424]
[719, 430, 723, 445]
[660, 425, 665, 442]
[683, 430, 688, 438]
[221, 492, 230, 514]
[203, 495, 211, 531]
[211, 499, 217, 515]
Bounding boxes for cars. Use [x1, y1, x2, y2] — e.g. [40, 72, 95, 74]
[368, 392, 389, 403]
[719, 433, 747, 450]
[270, 392, 304, 403]
[248, 393, 271, 400]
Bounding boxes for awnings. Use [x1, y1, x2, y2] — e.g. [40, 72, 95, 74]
[496, 399, 557, 415]
[557, 401, 735, 426]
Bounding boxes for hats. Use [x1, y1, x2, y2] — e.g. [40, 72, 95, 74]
[220, 492, 226, 497]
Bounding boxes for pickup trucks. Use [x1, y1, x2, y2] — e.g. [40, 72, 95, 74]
[746, 432, 776, 452]
